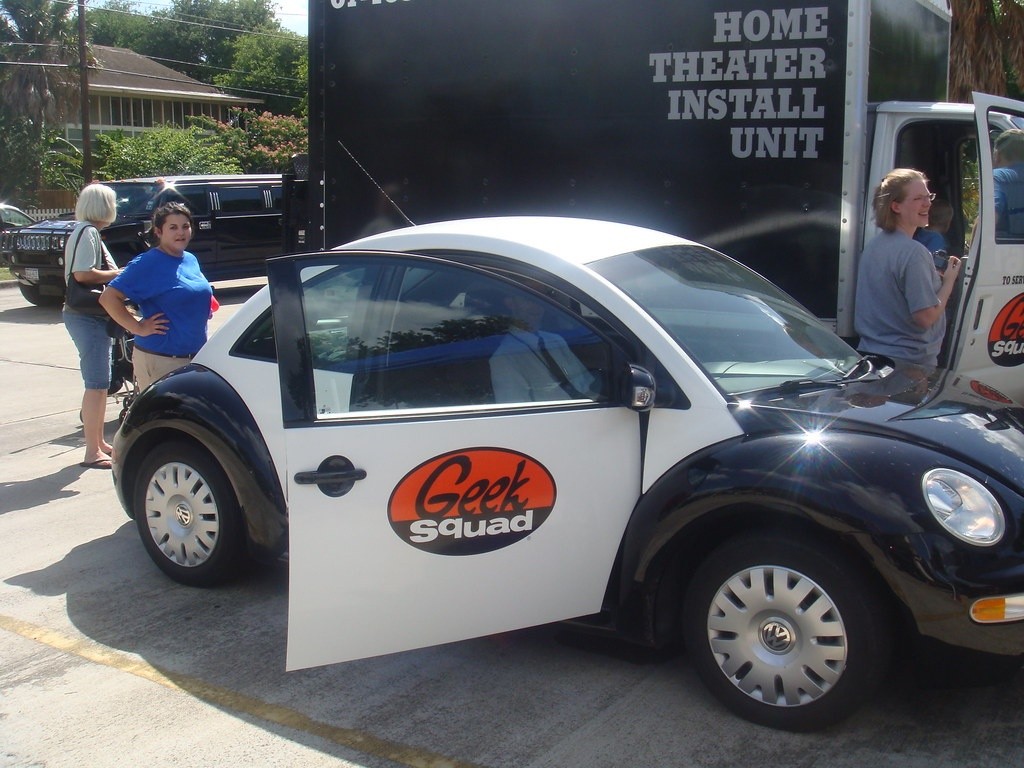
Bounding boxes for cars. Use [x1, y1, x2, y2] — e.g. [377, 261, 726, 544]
[0, 203, 40, 268]
[109, 217, 1023, 730]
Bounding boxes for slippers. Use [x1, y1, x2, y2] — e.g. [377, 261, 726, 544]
[81, 448, 112, 469]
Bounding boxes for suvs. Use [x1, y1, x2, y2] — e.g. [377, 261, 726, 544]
[4, 174, 281, 307]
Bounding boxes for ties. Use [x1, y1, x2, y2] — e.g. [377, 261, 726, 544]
[539, 339, 585, 399]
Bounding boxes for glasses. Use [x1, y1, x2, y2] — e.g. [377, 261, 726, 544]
[904, 193, 936, 202]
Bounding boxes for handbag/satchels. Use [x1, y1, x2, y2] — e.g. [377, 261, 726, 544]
[68, 224, 114, 315]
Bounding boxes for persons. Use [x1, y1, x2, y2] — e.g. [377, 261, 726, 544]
[489, 279, 597, 404]
[969, 129, 1024, 245]
[98, 203, 212, 393]
[852, 167, 961, 367]
[62, 183, 125, 469]
[914, 199, 953, 267]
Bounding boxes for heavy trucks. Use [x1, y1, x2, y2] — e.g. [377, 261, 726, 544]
[307, 0, 1023, 415]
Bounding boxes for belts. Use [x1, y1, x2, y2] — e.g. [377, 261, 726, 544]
[135, 344, 195, 359]
